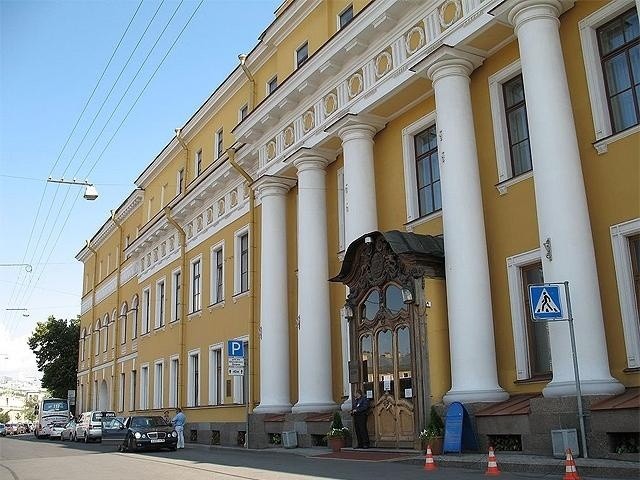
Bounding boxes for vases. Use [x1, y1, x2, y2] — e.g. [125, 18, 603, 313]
[330, 437, 344, 452]
[427, 438, 445, 457]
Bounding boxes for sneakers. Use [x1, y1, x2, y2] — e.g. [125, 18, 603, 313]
[353, 444, 369, 449]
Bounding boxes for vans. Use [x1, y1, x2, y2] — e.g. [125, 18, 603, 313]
[73, 411, 117, 444]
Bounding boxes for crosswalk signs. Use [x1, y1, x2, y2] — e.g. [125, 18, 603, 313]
[530, 284, 562, 321]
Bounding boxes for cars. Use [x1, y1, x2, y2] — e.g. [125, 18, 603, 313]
[48, 420, 69, 441]
[100, 415, 179, 454]
[0, 420, 35, 437]
[107, 416, 130, 430]
[59, 415, 80, 441]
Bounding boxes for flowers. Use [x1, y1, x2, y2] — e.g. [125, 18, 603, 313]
[326, 425, 350, 438]
[420, 426, 441, 439]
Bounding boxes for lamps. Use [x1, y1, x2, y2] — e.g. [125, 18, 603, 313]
[46, 175, 100, 202]
[343, 300, 354, 320]
[6, 308, 31, 317]
[401, 285, 415, 304]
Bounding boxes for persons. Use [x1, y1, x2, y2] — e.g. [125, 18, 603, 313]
[171, 408, 186, 450]
[163, 410, 171, 426]
[351, 389, 370, 449]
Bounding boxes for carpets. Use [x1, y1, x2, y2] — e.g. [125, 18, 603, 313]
[311, 447, 422, 461]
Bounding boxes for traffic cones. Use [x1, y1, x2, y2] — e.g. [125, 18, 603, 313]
[422, 445, 439, 472]
[484, 446, 503, 476]
[562, 447, 580, 480]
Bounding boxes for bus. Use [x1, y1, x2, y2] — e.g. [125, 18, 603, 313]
[34, 396, 72, 440]
